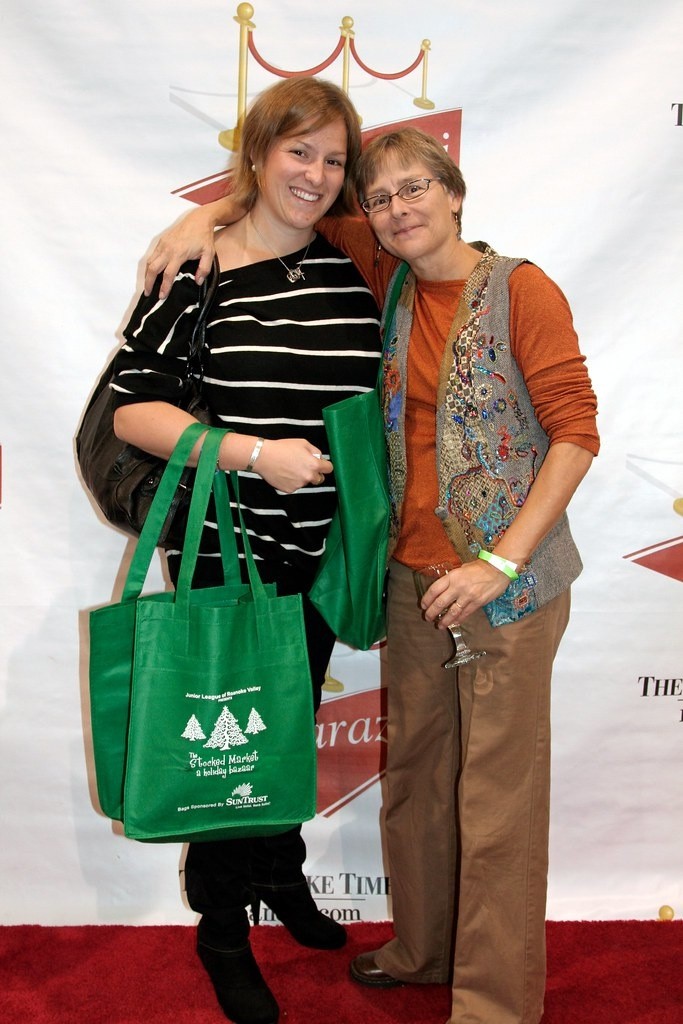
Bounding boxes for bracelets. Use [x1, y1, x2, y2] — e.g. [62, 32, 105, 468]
[216, 458, 221, 473]
[244, 436, 264, 471]
[478, 549, 520, 582]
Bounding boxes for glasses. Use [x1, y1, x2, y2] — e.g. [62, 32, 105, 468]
[359, 176, 446, 214]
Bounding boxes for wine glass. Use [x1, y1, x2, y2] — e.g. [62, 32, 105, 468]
[321, 658, 344, 692]
[414, 561, 486, 669]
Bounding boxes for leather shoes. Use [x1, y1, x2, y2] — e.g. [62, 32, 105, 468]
[350, 950, 408, 987]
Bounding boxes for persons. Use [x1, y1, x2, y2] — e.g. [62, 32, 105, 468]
[108, 74, 382, 1023]
[143, 126, 601, 1023]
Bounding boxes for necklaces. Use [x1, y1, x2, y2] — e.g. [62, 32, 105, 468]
[248, 208, 315, 284]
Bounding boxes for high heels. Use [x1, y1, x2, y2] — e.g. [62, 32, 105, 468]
[251, 877, 348, 951]
[195, 936, 279, 1024]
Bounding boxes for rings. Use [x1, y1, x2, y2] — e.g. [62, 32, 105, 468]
[455, 601, 465, 610]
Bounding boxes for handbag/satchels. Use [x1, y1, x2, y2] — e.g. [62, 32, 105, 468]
[85, 424, 316, 841]
[308, 256, 410, 650]
[76, 249, 220, 547]
